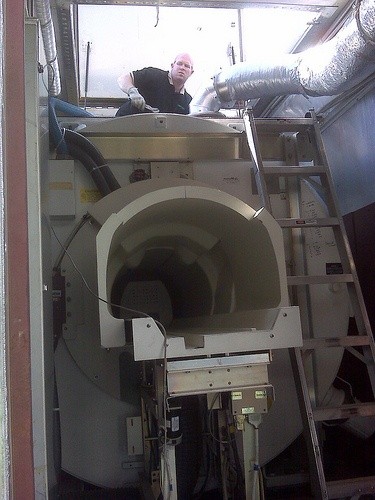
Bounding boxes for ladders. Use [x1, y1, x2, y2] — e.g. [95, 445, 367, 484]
[242, 106, 374, 500]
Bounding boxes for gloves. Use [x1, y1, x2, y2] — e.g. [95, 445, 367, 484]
[127, 86, 145, 112]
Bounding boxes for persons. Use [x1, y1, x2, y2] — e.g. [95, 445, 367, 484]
[114, 53, 195, 117]
